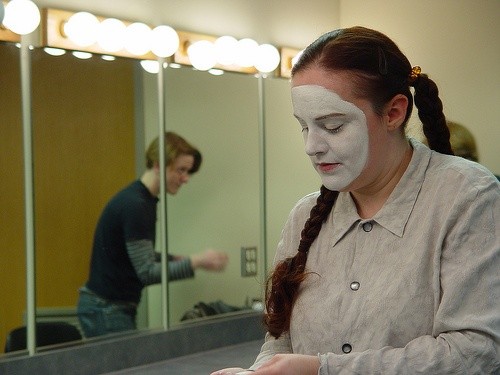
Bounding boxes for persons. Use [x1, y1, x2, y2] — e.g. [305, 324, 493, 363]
[421, 120, 500, 180]
[210, 25, 500, 375]
[79, 132, 228, 339]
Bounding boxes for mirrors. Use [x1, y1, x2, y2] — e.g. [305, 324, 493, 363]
[0, 41, 322, 359]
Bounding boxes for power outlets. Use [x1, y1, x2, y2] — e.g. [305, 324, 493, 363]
[239, 245, 258, 278]
